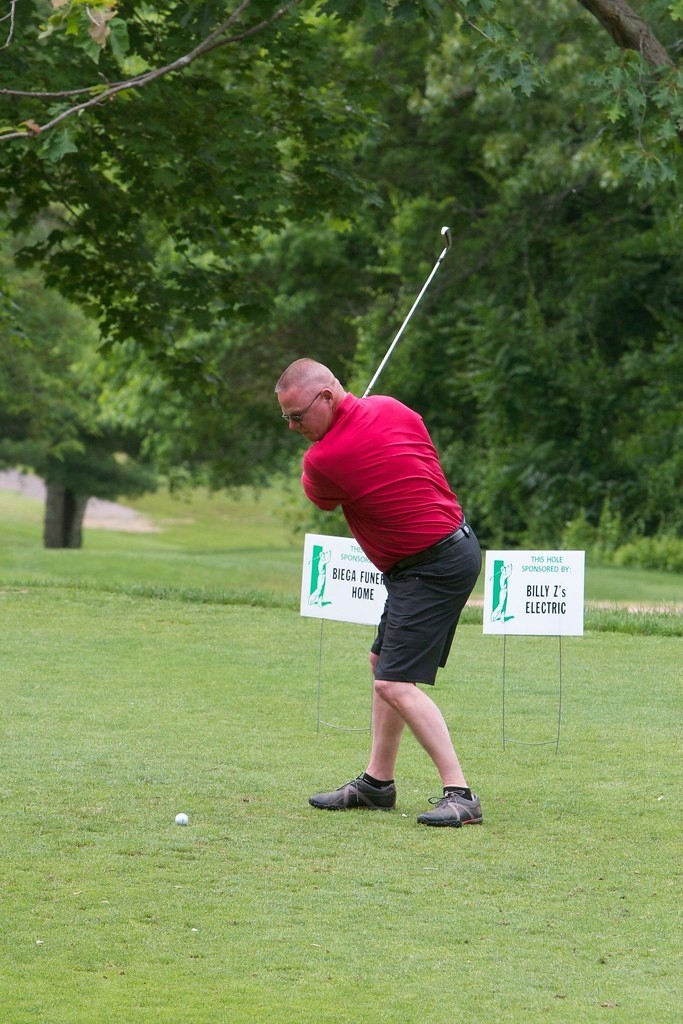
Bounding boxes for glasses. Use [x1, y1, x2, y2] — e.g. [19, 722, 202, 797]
[282, 392, 322, 423]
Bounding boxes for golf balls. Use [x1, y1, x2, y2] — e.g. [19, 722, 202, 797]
[176, 813, 189, 825]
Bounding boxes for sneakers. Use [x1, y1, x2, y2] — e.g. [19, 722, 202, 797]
[309, 774, 396, 810]
[416, 791, 483, 827]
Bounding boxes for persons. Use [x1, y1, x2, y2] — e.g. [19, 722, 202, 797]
[276, 358, 484, 827]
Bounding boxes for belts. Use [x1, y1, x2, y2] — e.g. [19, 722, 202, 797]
[395, 524, 470, 568]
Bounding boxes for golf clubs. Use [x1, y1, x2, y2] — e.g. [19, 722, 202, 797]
[362, 226, 451, 398]
